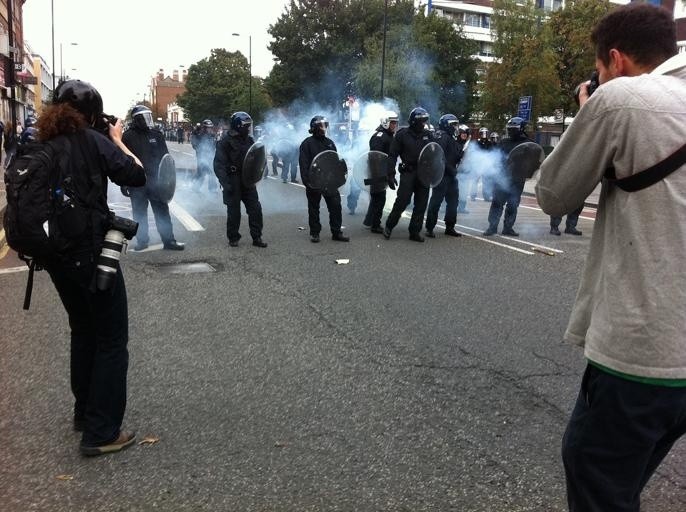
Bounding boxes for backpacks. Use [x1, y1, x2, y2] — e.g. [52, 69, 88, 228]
[3, 128, 102, 311]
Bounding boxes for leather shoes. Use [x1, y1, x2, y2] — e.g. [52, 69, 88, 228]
[332, 234, 349, 241]
[565, 227, 582, 236]
[252, 240, 266, 247]
[550, 228, 560, 235]
[362, 218, 461, 242]
[483, 227, 496, 236]
[229, 240, 237, 246]
[310, 233, 318, 241]
[134, 243, 147, 250]
[502, 228, 519, 236]
[163, 241, 184, 250]
[263, 174, 297, 183]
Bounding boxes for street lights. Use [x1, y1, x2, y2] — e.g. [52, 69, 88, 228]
[230, 31, 253, 115]
[60, 41, 79, 83]
[127, 91, 147, 110]
[63, 67, 77, 81]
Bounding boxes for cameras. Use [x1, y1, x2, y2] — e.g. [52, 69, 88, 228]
[574, 71, 599, 108]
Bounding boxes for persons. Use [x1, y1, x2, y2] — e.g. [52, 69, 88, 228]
[34, 79, 148, 456]
[533, 5, 686, 512]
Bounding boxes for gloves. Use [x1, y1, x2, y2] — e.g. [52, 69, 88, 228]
[120, 186, 132, 197]
[387, 173, 397, 189]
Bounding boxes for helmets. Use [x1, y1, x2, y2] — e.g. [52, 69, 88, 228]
[309, 115, 330, 136]
[202, 119, 214, 134]
[379, 106, 526, 143]
[52, 80, 102, 122]
[130, 104, 155, 128]
[228, 111, 253, 137]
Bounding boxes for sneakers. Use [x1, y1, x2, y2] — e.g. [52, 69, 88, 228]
[78, 431, 136, 456]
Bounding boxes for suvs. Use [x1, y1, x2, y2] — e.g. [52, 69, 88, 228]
[331, 121, 347, 145]
